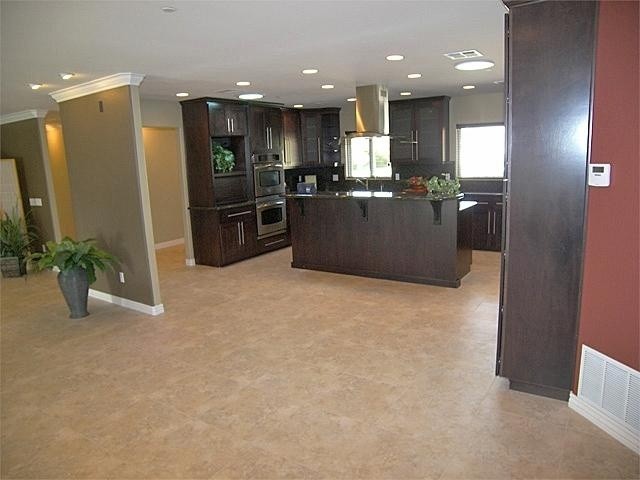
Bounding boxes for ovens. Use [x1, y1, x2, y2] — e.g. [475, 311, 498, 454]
[253, 166, 285, 199]
[253, 194, 289, 240]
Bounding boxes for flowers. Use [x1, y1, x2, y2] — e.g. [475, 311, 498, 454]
[404, 176, 427, 185]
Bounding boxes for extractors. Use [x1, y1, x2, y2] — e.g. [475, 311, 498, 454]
[336, 86, 394, 139]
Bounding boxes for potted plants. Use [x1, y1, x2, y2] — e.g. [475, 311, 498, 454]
[212, 145, 236, 174]
[0, 197, 42, 277]
[24, 237, 124, 320]
[425, 176, 461, 199]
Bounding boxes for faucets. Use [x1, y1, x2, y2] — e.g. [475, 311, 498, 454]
[356, 177, 368, 190]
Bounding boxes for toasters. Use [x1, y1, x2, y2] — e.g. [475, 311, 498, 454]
[297, 182, 316, 194]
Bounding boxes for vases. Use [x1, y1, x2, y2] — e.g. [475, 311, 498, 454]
[405, 187, 427, 196]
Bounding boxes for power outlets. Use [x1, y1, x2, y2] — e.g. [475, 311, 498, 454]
[29, 198, 36, 206]
[588, 163, 612, 188]
[119, 272, 125, 283]
[35, 198, 42, 206]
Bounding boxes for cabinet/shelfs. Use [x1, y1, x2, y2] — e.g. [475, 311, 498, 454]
[285, 190, 480, 288]
[464, 193, 503, 251]
[495, 0, 597, 401]
[388, 95, 452, 164]
[178, 96, 344, 268]
